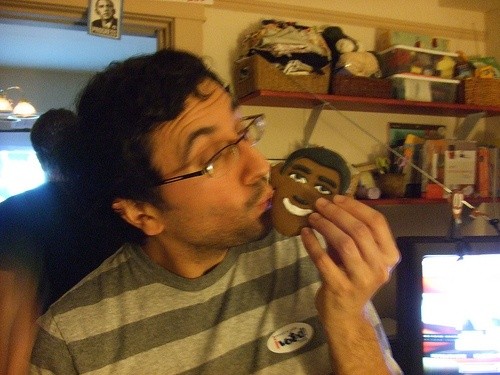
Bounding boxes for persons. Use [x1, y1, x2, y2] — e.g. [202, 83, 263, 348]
[30, 44, 408, 375]
[0, 108, 146, 323]
[269, 146, 353, 236]
[92, 0, 119, 34]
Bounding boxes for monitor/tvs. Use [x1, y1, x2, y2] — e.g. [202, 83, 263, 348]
[394, 234, 500, 375]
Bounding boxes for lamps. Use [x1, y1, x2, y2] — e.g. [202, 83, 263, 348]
[0, 86, 39, 123]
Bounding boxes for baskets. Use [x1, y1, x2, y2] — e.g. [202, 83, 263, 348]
[231, 53, 332, 100]
[455, 76, 500, 108]
[331, 74, 395, 100]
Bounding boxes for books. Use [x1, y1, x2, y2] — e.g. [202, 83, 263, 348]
[387, 123, 500, 201]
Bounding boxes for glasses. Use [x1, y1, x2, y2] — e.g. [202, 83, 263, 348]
[139, 112, 266, 189]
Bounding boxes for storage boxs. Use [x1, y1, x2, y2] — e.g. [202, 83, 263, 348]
[232, 44, 460, 104]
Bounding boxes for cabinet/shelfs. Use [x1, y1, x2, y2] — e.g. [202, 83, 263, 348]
[234, 89, 500, 208]
